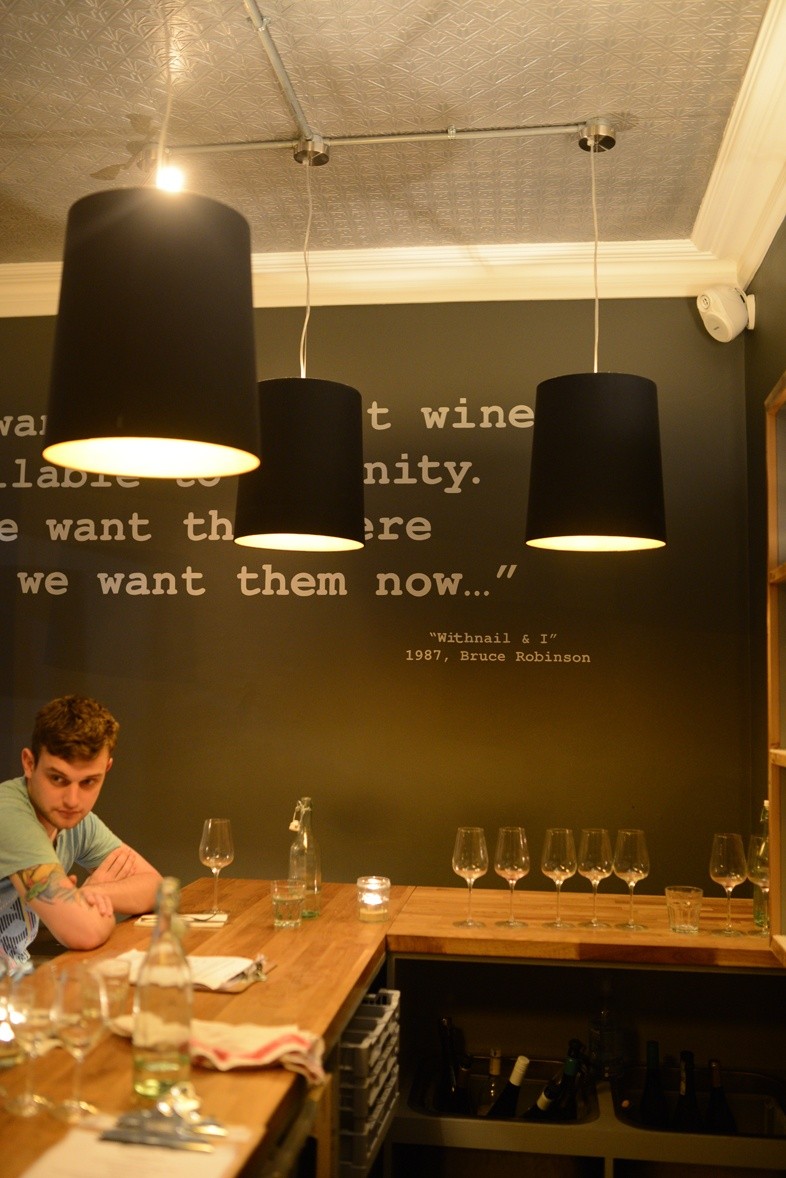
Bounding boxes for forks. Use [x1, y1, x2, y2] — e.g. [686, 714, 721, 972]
[140, 912, 216, 922]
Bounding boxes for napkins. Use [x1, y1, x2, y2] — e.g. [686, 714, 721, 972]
[134, 914, 229, 928]
[21, 1111, 251, 1178]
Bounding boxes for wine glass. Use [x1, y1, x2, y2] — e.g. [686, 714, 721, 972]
[199, 818, 235, 916]
[709, 832, 747, 937]
[540, 827, 577, 930]
[747, 834, 771, 939]
[7, 952, 111, 1125]
[613, 827, 651, 932]
[493, 825, 530, 927]
[577, 827, 613, 931]
[451, 826, 489, 929]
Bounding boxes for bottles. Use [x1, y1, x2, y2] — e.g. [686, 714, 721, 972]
[434, 994, 737, 1136]
[752, 800, 770, 928]
[0, 942, 27, 1067]
[288, 796, 321, 918]
[130, 878, 194, 1098]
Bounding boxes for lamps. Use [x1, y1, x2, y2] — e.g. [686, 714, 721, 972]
[525, 126, 666, 552]
[234, 142, 364, 551]
[42, 0, 261, 478]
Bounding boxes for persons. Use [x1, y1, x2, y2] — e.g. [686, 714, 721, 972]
[0, 696, 162, 983]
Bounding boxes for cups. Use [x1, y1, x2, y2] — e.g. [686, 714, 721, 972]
[356, 874, 392, 922]
[269, 880, 306, 928]
[80, 957, 131, 1031]
[664, 886, 703, 933]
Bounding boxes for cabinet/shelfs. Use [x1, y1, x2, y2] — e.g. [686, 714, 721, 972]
[763, 373, 786, 969]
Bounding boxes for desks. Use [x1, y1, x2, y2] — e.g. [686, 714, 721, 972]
[0, 878, 786, 1178]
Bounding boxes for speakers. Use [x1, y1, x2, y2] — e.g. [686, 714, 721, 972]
[693, 282, 755, 343]
